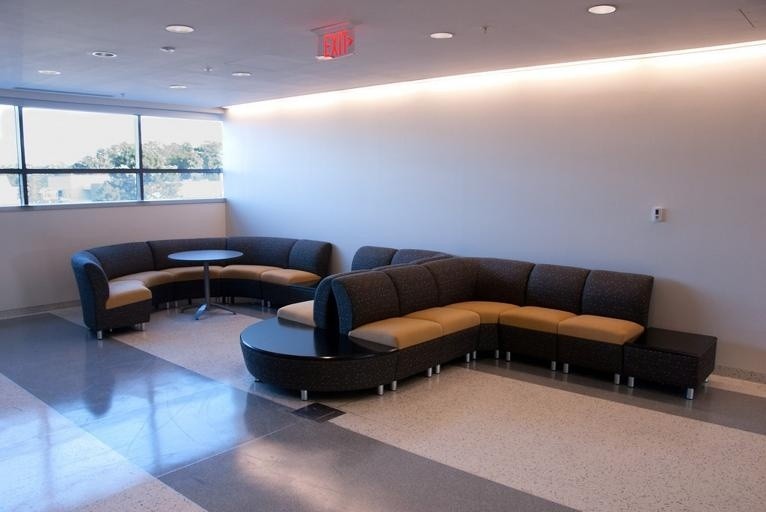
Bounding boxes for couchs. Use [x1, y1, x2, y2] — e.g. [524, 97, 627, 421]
[68, 234, 336, 342]
[167, 248, 244, 320]
[238, 244, 718, 403]
